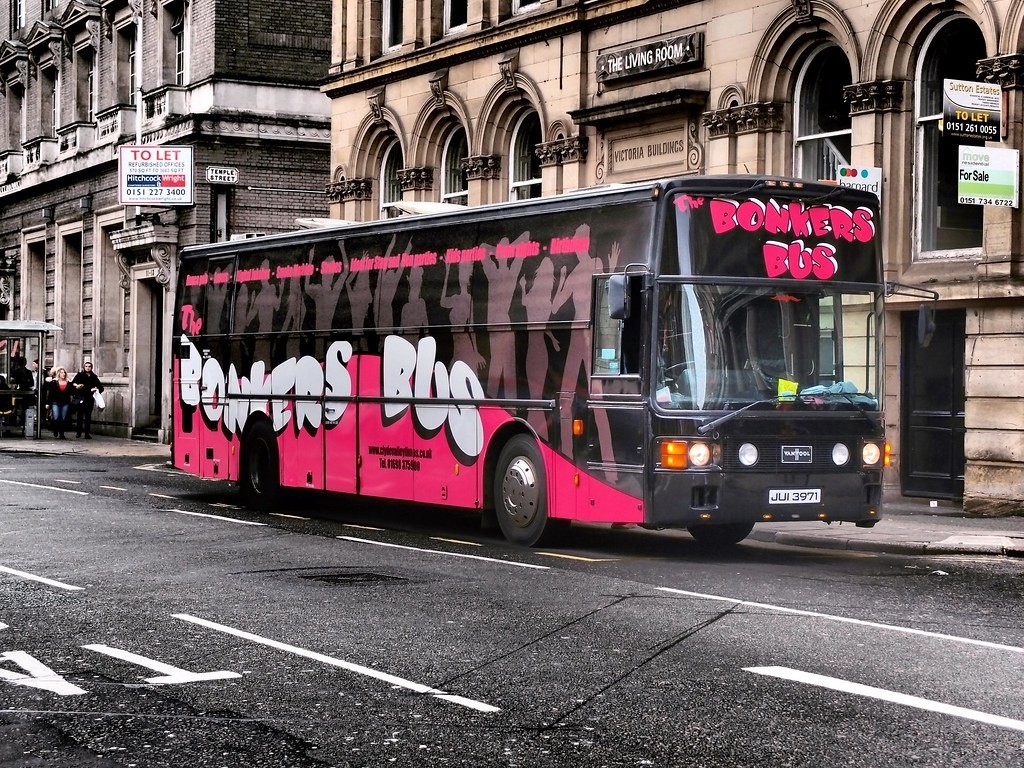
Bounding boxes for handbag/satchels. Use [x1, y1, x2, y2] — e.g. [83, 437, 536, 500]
[93, 389, 105, 412]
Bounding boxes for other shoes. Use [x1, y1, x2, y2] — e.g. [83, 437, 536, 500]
[76, 434, 81, 438]
[54, 431, 58, 437]
[85, 434, 93, 439]
[59, 434, 67, 440]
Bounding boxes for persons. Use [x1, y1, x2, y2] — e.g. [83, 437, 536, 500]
[71, 362, 104, 438]
[9, 357, 34, 389]
[31, 359, 57, 430]
[43, 368, 84, 439]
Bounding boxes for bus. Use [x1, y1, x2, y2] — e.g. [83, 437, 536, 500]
[171, 174, 939, 547]
